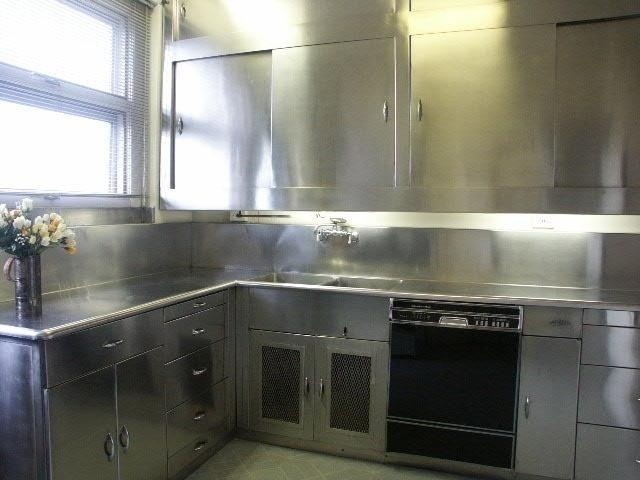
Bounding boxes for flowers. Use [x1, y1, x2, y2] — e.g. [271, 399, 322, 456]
[0, 197, 76, 258]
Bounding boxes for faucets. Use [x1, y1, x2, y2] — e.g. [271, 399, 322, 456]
[313, 218, 359, 244]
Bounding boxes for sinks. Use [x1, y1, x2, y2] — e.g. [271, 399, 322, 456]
[321, 277, 396, 291]
[246, 273, 332, 286]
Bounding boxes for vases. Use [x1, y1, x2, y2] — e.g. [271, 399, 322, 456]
[3, 253, 42, 320]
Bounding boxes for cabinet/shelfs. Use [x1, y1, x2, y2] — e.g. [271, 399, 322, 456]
[237, 287, 389, 463]
[0, 307, 167, 480]
[164, 289, 237, 480]
[514, 304, 583, 479]
[158, 0, 400, 210]
[575, 309, 640, 480]
[400, 0, 640, 214]
